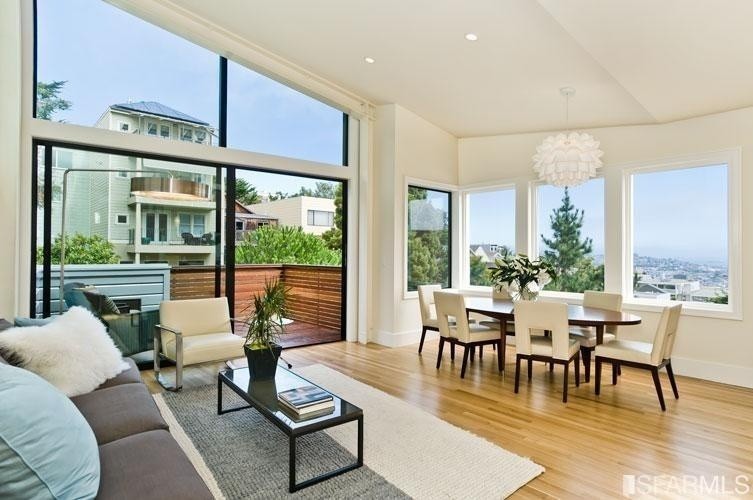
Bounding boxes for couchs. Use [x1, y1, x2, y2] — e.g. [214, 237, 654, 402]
[0, 319, 216, 500]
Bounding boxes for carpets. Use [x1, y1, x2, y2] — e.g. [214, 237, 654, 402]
[152, 351, 546, 500]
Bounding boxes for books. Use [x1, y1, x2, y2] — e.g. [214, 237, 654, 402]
[275, 383, 335, 424]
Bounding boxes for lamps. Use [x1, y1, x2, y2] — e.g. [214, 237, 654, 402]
[532, 86, 603, 187]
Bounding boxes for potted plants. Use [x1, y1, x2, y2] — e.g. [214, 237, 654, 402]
[224, 274, 295, 380]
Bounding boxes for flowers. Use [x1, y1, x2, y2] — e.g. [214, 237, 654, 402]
[482, 250, 562, 300]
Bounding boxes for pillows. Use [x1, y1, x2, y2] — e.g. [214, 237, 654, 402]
[0, 361, 101, 500]
[11, 312, 62, 326]
[15, 314, 60, 326]
[0, 305, 132, 398]
[83, 289, 121, 314]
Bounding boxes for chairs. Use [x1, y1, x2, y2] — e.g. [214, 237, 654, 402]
[154, 296, 247, 392]
[62, 282, 159, 356]
[417, 279, 681, 413]
